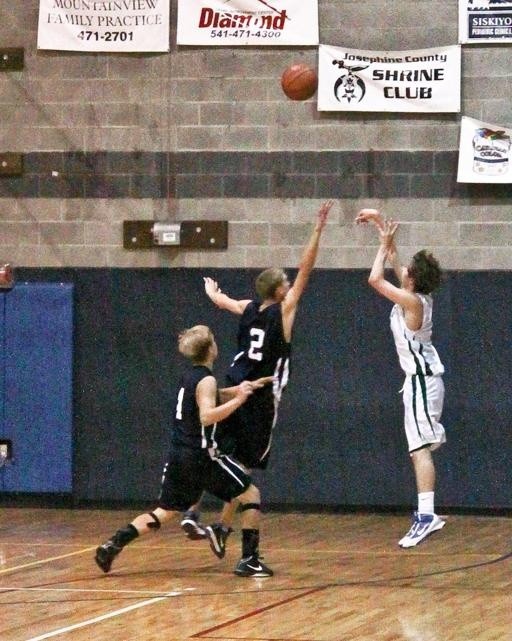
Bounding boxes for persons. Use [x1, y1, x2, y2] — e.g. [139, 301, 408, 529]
[93, 325, 280, 580]
[177, 197, 337, 557]
[354, 208, 449, 548]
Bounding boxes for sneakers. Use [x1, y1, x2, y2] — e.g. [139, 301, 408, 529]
[398, 510, 448, 550]
[234, 555, 275, 578]
[180, 510, 234, 559]
[94, 539, 123, 574]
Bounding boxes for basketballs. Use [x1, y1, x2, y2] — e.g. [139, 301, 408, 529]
[282, 63, 318, 101]
